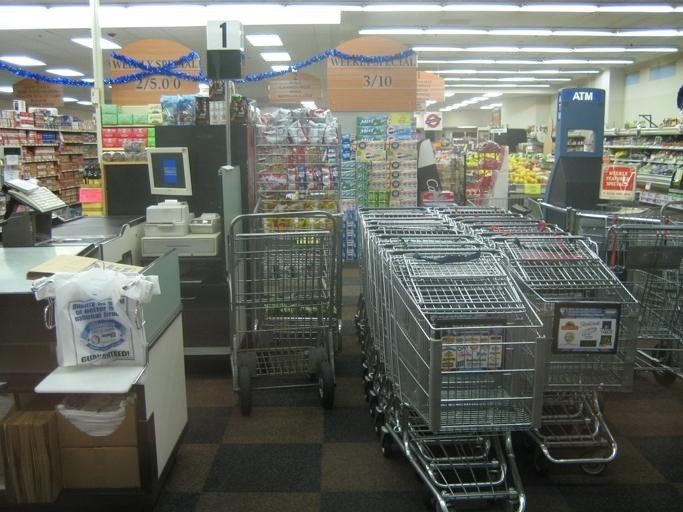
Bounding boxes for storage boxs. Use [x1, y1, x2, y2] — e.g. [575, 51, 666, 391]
[57, 446, 143, 490]
[57, 393, 138, 447]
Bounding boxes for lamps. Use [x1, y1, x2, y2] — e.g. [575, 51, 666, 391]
[0, 0, 682, 98]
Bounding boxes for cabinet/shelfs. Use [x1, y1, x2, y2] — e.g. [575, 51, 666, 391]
[464, 125, 683, 209]
[0, 126, 101, 207]
[251, 121, 343, 322]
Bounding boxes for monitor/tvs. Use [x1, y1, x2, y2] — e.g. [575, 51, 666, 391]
[146, 147, 192, 197]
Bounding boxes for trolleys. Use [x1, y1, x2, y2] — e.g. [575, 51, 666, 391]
[226, 211, 340, 415]
[350, 198, 683, 512]
